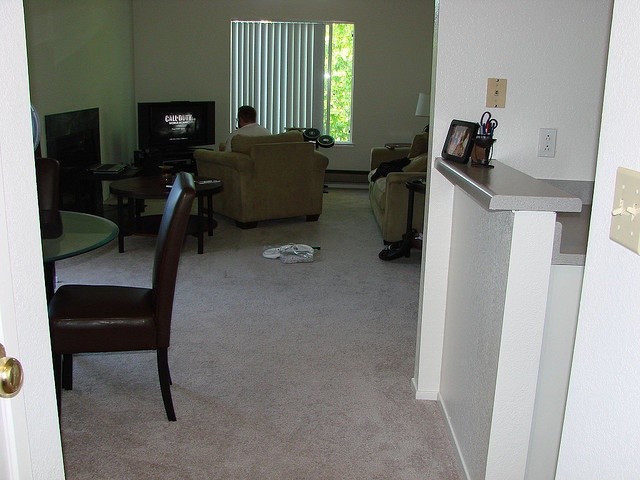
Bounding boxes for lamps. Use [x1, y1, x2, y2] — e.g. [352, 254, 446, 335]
[49, 172, 197, 422]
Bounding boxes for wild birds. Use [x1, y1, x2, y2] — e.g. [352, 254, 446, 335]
[93, 164, 127, 174]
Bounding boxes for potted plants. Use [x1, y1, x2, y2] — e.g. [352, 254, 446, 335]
[441, 118, 478, 164]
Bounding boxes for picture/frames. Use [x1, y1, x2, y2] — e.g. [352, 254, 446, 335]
[143, 148, 214, 179]
[93, 162, 143, 237]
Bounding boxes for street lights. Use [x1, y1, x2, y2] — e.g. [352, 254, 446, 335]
[378, 229, 418, 262]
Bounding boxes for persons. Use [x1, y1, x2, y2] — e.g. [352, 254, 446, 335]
[219, 106, 270, 153]
[453, 129, 469, 155]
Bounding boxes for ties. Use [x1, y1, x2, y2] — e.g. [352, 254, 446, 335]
[139, 99, 217, 158]
[44, 107, 101, 168]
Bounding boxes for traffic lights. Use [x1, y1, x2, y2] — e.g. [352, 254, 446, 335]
[401, 152, 426, 172]
[405, 132, 428, 159]
[231, 130, 306, 156]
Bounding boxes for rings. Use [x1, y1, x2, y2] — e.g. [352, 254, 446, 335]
[477, 122, 495, 137]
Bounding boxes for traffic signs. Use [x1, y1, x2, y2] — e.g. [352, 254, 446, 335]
[607, 166, 640, 256]
[536, 127, 556, 158]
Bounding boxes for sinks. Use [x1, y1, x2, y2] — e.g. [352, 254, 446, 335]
[193, 130, 329, 230]
[368, 146, 426, 245]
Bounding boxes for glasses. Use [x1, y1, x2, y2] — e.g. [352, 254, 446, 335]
[414, 92, 430, 118]
